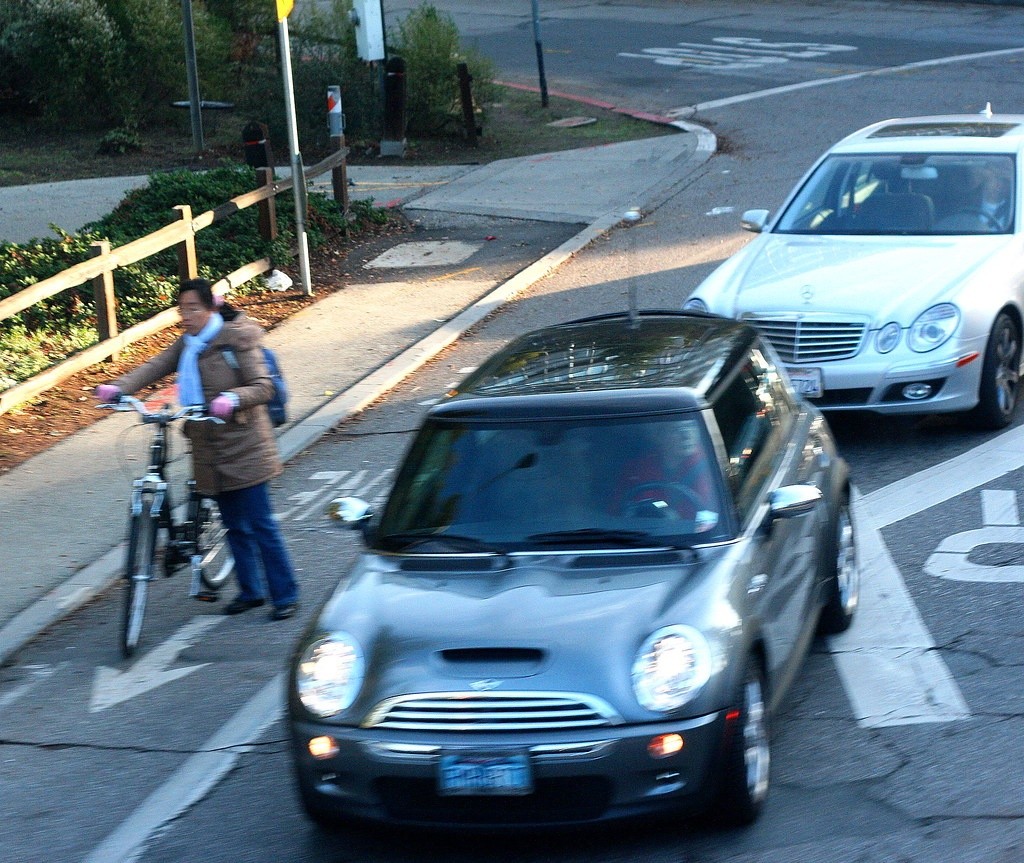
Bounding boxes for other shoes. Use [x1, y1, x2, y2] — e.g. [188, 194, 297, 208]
[271, 603, 300, 618]
[224, 598, 264, 615]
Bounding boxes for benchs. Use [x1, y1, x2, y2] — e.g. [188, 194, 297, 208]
[886, 163, 983, 216]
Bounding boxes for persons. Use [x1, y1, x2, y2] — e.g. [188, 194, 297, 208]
[94, 277, 300, 620]
[609, 419, 717, 524]
[943, 162, 1011, 229]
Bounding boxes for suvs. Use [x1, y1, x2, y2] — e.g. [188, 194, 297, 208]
[283, 301, 857, 861]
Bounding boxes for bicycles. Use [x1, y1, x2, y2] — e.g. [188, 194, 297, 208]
[93, 380, 236, 660]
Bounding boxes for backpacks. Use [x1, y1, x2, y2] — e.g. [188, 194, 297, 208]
[258, 348, 287, 428]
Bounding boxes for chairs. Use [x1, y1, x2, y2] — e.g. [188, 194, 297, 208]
[857, 173, 935, 230]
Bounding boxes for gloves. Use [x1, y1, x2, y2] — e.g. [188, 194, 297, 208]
[211, 397, 233, 416]
[96, 384, 123, 402]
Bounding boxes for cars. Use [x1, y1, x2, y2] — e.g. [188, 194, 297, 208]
[681, 99, 1023, 430]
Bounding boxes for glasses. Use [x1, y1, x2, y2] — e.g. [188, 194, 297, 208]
[177, 305, 204, 316]
